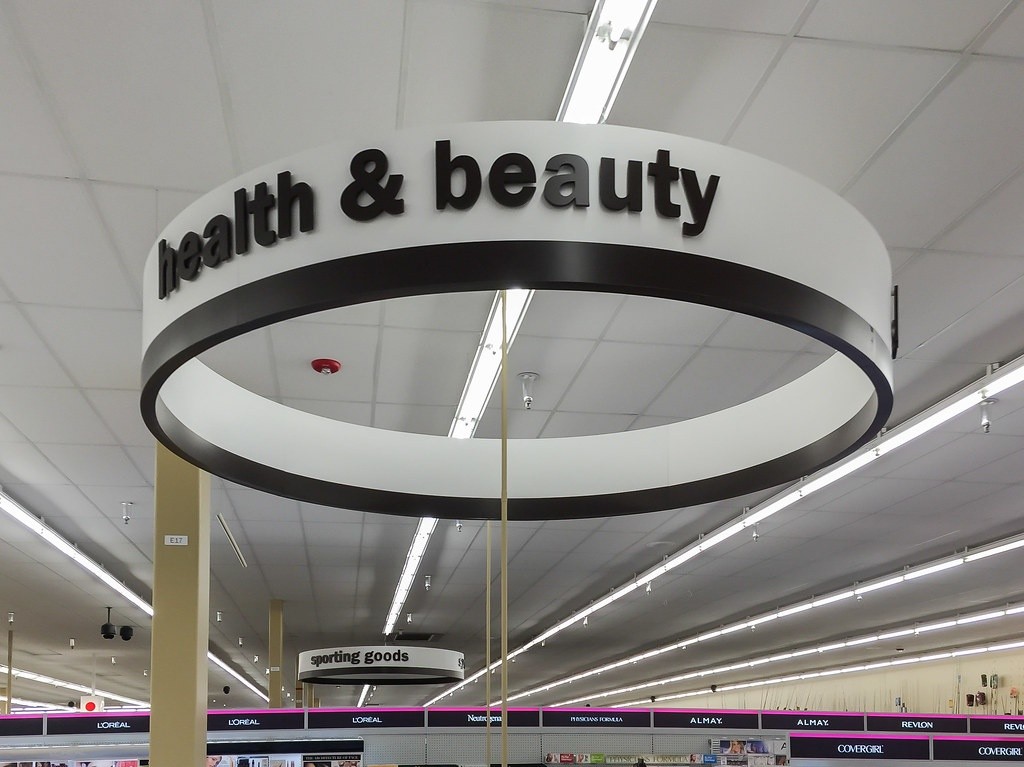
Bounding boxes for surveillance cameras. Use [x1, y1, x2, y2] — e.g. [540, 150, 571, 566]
[120, 626, 134, 642]
[100, 623, 115, 639]
[223, 686, 231, 694]
